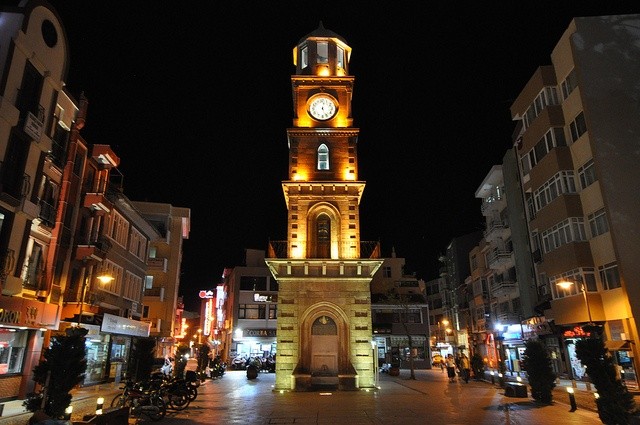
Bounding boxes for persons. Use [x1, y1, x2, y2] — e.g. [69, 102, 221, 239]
[458, 350, 473, 385]
[444, 352, 457, 386]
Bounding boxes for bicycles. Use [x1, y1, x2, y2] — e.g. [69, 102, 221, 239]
[111, 371, 200, 423]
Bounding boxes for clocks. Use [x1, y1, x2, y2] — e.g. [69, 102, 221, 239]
[304, 93, 341, 123]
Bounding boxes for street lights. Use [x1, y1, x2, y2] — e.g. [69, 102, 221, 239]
[446, 328, 452, 334]
[77, 270, 115, 326]
[442, 319, 459, 377]
[555, 277, 592, 323]
